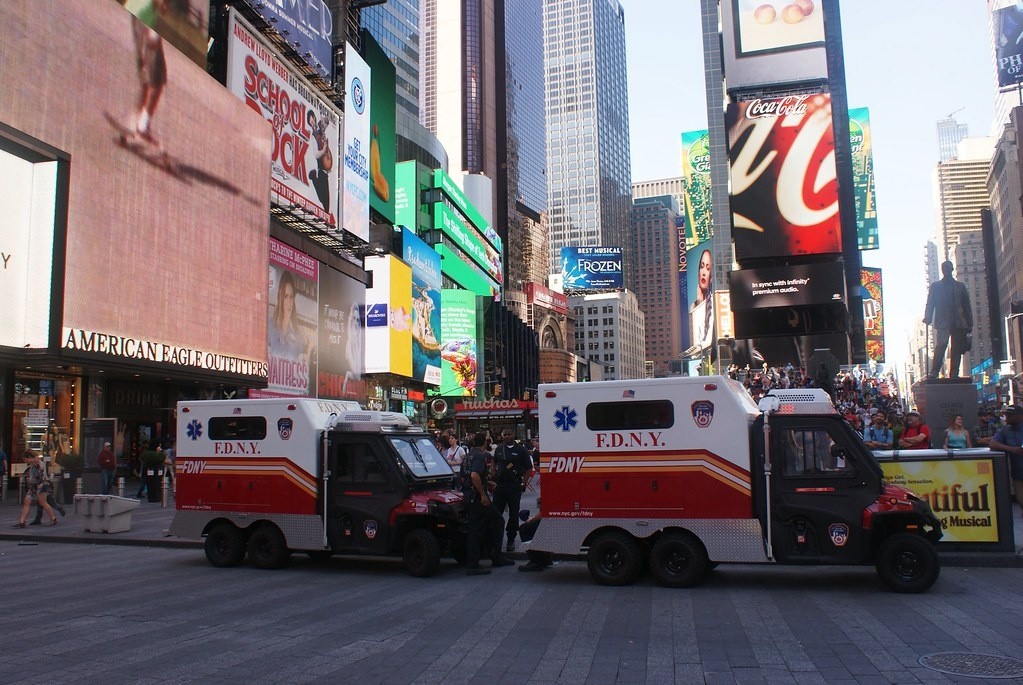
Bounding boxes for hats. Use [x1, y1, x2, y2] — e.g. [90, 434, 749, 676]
[1003, 404, 1023, 414]
[906, 410, 921, 416]
[104, 442, 111, 447]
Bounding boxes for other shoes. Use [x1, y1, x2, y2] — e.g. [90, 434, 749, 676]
[12, 522, 25, 528]
[59, 506, 66, 516]
[30, 520, 41, 525]
[465, 566, 491, 575]
[136, 492, 145, 498]
[47, 517, 57, 526]
[519, 563, 530, 571]
[492, 559, 515, 567]
[544, 560, 553, 568]
[506, 542, 516, 550]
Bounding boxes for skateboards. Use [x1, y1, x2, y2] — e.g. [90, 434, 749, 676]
[102, 113, 188, 175]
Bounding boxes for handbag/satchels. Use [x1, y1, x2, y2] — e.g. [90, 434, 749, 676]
[133, 459, 143, 481]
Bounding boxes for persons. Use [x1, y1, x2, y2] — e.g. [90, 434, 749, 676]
[123, 14, 167, 145]
[974, 405, 1023, 509]
[132, 440, 176, 498]
[307, 109, 333, 214]
[11, 451, 66, 529]
[345, 302, 361, 381]
[0, 444, 8, 496]
[898, 410, 930, 450]
[490, 426, 553, 572]
[863, 410, 894, 450]
[943, 414, 972, 449]
[434, 429, 540, 477]
[689, 250, 714, 350]
[96, 441, 116, 495]
[727, 354, 901, 473]
[460, 433, 516, 575]
[268, 270, 304, 358]
[923, 261, 973, 378]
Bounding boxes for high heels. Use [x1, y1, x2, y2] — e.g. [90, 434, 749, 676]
[172, 492, 176, 500]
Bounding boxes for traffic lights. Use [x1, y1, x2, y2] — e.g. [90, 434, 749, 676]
[983, 375, 989, 385]
[911, 407, 918, 412]
[900, 383, 903, 390]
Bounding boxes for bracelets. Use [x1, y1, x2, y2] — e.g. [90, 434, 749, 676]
[5, 470, 8, 472]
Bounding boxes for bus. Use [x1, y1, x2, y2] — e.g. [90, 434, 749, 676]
[451, 399, 538, 448]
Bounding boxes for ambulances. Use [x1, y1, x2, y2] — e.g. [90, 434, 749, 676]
[165, 396, 477, 577]
[519, 375, 946, 595]
